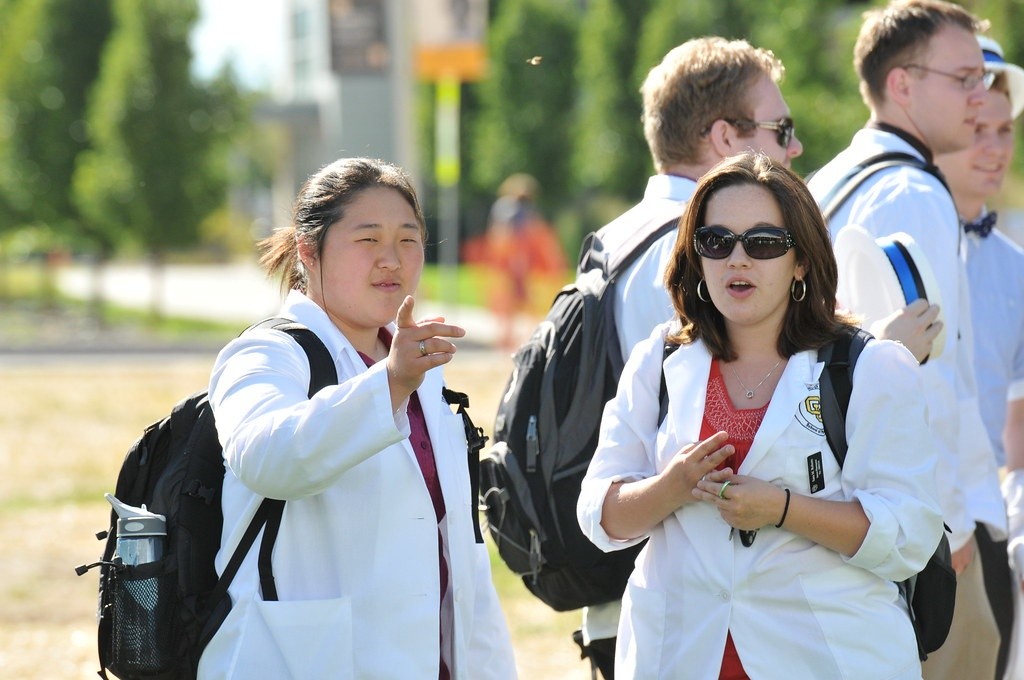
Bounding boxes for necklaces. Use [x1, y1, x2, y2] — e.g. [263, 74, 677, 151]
[728, 348, 785, 399]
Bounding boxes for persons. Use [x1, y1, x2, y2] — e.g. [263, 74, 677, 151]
[797, 0, 1008, 678]
[482, 173, 570, 351]
[574, 147, 945, 680]
[929, 34, 1024, 604]
[479, 36, 941, 680]
[192, 155, 528, 680]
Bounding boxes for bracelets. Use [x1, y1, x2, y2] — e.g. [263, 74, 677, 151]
[775, 488, 791, 528]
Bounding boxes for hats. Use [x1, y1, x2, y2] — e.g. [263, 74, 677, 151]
[975, 36, 1024, 122]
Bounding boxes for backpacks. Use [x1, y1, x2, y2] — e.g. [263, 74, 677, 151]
[75, 317, 338, 680]
[661, 324, 957, 661]
[477, 218, 695, 610]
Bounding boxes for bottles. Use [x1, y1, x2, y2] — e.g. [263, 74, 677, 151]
[104, 490, 164, 674]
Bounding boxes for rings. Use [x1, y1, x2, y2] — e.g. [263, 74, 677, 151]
[718, 480, 732, 500]
[418, 340, 428, 357]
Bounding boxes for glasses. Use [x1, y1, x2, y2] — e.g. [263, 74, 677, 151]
[700, 118, 794, 147]
[692, 225, 797, 260]
[904, 64, 995, 90]
